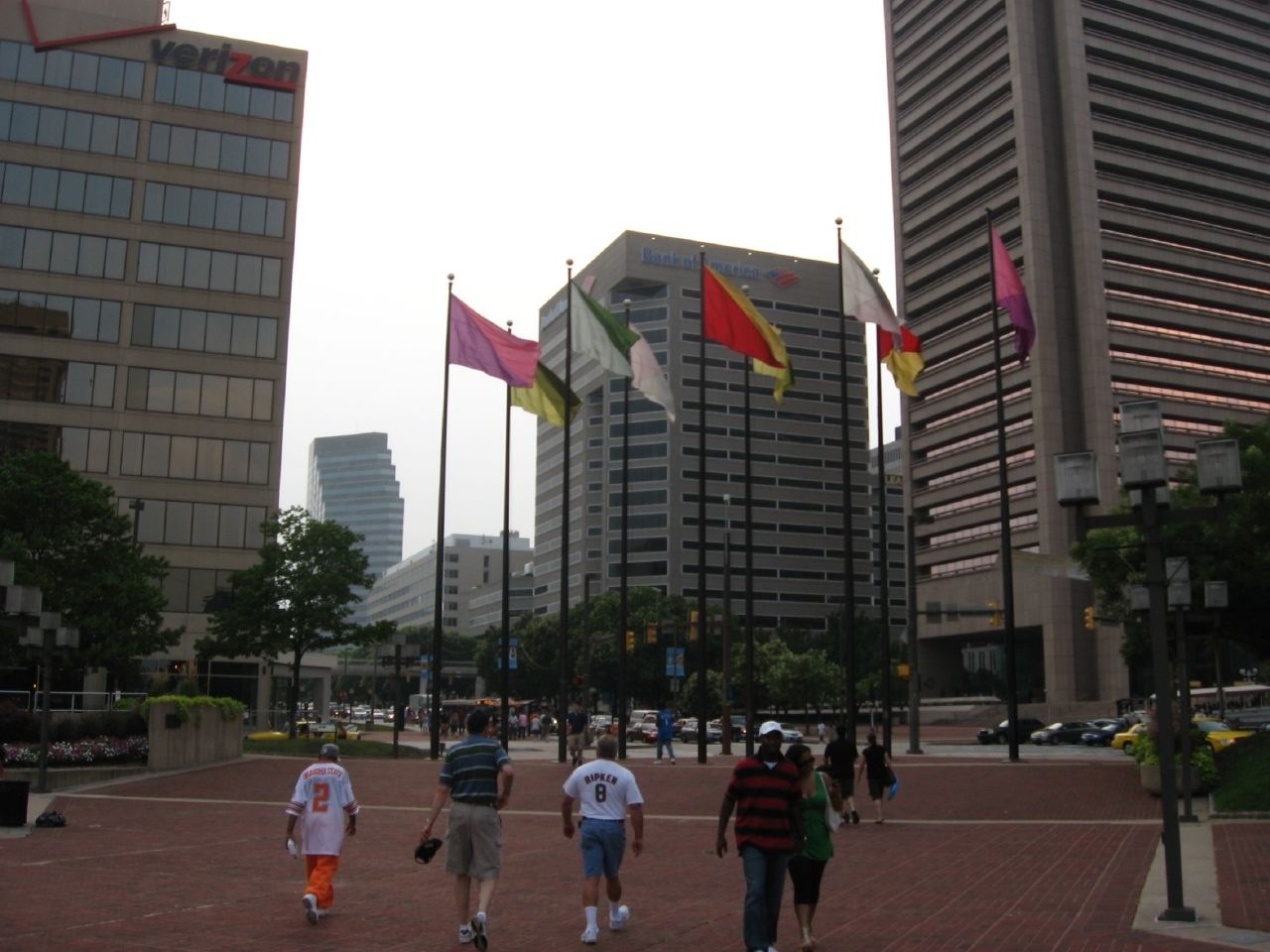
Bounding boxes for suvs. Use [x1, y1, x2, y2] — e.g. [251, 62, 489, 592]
[976, 717, 1046, 745]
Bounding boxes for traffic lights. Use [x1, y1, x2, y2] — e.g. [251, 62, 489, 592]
[1084, 605, 1094, 631]
[625, 630, 635, 652]
[685, 609, 700, 643]
[646, 619, 657, 644]
[1052, 398, 1244, 927]
[987, 599, 1000, 628]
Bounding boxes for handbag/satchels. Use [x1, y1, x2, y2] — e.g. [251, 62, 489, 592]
[36, 812, 66, 827]
[888, 782, 899, 799]
[819, 772, 841, 833]
[884, 766, 896, 786]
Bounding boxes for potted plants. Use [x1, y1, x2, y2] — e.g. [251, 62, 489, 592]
[1133, 728, 1222, 800]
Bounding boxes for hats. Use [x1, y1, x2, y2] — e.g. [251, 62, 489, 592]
[758, 720, 784, 738]
[414, 839, 442, 864]
[320, 744, 340, 757]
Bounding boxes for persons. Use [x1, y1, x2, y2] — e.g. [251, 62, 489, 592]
[554, 735, 646, 943]
[567, 701, 587, 766]
[652, 704, 677, 764]
[821, 724, 865, 823]
[403, 706, 433, 732]
[285, 743, 358, 926]
[857, 732, 898, 825]
[439, 705, 553, 740]
[413, 710, 515, 951]
[715, 720, 804, 952]
[785, 743, 834, 948]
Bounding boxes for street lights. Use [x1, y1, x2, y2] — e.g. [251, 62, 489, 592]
[128, 497, 145, 546]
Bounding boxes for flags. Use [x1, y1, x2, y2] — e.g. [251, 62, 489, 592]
[621, 299, 678, 424]
[738, 282, 793, 408]
[564, 260, 642, 380]
[835, 218, 902, 352]
[504, 321, 582, 429]
[446, 274, 541, 389]
[872, 269, 923, 402]
[985, 208, 1036, 365]
[698, 246, 787, 369]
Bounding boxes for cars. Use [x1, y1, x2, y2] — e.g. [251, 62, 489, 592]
[296, 701, 419, 724]
[1081, 711, 1147, 747]
[1111, 713, 1257, 761]
[777, 722, 804, 743]
[589, 709, 760, 744]
[1029, 721, 1099, 746]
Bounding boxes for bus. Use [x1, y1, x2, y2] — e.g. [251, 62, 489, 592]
[441, 698, 540, 735]
[1143, 683, 1270, 735]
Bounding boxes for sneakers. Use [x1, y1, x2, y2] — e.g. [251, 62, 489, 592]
[581, 928, 599, 942]
[319, 908, 329, 916]
[303, 893, 319, 925]
[471, 918, 489, 951]
[609, 905, 629, 930]
[459, 929, 476, 944]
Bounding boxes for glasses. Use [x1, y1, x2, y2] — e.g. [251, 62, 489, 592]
[799, 757, 815, 767]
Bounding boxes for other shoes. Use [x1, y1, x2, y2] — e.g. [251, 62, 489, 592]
[802, 942, 814, 952]
[654, 760, 661, 763]
[672, 758, 676, 763]
[573, 757, 577, 763]
[852, 811, 860, 824]
[843, 813, 850, 823]
[579, 761, 583, 765]
